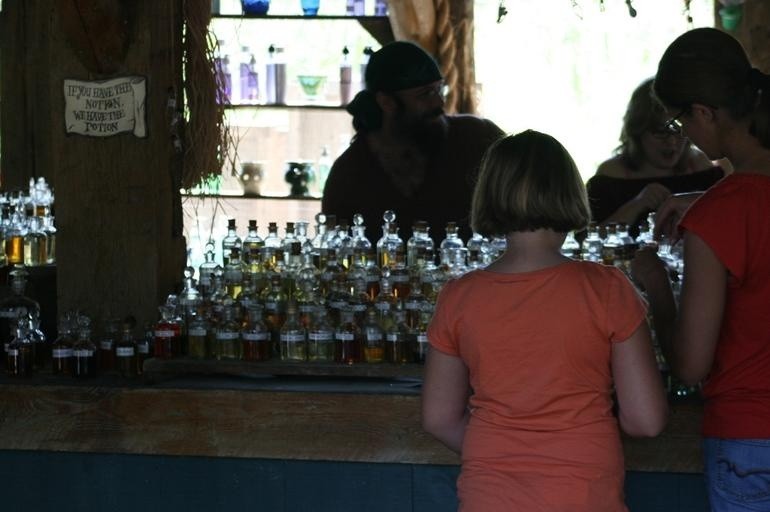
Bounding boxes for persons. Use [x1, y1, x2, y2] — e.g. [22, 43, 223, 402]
[418, 126, 676, 511]
[628, 27, 769, 511]
[318, 39, 509, 268]
[586, 71, 725, 231]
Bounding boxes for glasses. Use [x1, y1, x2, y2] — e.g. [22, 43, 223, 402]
[663, 102, 718, 136]
[397, 84, 445, 100]
[648, 127, 668, 139]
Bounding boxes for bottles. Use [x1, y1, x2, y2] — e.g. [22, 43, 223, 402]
[0, 295, 185, 380]
[318, 144, 333, 194]
[0, 174, 54, 294]
[181, 210, 505, 363]
[203, 0, 387, 105]
[207, 172, 221, 194]
[561, 220, 684, 367]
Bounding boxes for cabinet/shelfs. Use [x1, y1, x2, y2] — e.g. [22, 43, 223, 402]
[180, 0, 392, 282]
